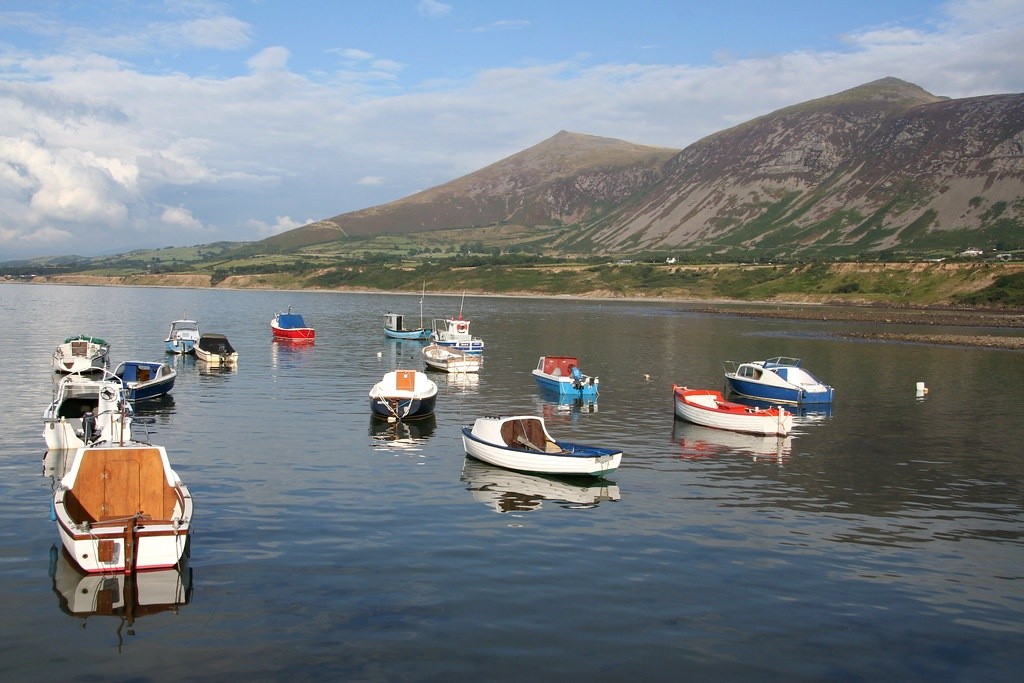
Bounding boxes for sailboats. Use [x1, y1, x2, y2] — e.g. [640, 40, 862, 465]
[426, 290, 485, 357]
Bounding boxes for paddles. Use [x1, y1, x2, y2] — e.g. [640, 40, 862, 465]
[707, 388, 727, 411]
[517, 436, 546, 454]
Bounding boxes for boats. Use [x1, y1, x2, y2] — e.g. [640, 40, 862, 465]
[532, 358, 600, 399]
[673, 419, 794, 469]
[194, 333, 239, 363]
[42, 366, 135, 450]
[460, 415, 622, 476]
[100, 360, 178, 403]
[419, 341, 483, 375]
[460, 454, 623, 515]
[366, 369, 439, 421]
[719, 357, 836, 407]
[382, 312, 433, 341]
[269, 307, 315, 344]
[50, 335, 110, 377]
[164, 310, 208, 356]
[51, 542, 190, 656]
[50, 443, 195, 572]
[671, 382, 794, 436]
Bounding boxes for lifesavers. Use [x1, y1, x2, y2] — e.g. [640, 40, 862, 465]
[457, 322, 468, 334]
